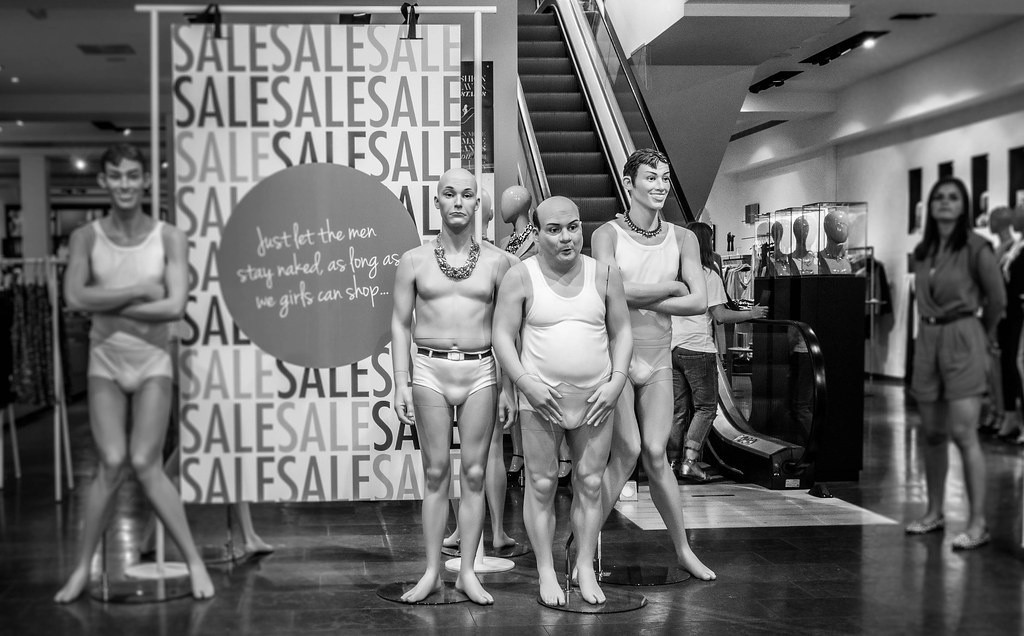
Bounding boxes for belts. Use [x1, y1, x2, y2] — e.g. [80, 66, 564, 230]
[921, 311, 974, 325]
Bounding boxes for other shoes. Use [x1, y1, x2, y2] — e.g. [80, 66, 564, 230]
[670, 461, 682, 476]
[952, 532, 990, 547]
[906, 515, 944, 532]
[679, 459, 712, 481]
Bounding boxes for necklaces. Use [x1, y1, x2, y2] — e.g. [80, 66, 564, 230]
[504, 221, 534, 253]
[623, 209, 663, 238]
[434, 233, 481, 280]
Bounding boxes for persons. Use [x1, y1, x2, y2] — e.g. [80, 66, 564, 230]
[391, 147, 718, 608]
[667, 221, 769, 482]
[52, 148, 216, 604]
[138, 441, 275, 554]
[971, 188, 1024, 444]
[905, 176, 1004, 549]
[908, 201, 923, 237]
[766, 209, 853, 276]
